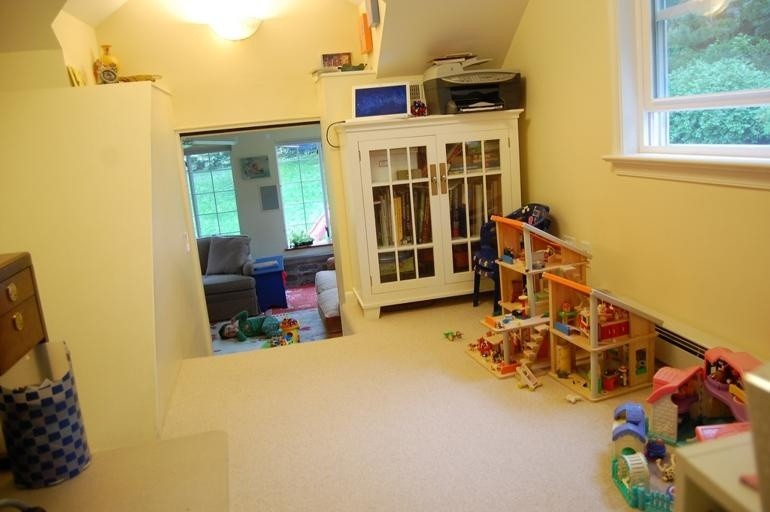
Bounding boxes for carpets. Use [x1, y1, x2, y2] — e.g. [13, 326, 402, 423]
[209, 281, 344, 354]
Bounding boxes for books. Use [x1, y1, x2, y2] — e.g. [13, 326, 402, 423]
[459, 101, 504, 113]
[374, 138, 505, 283]
[443, 51, 473, 57]
[462, 56, 491, 68]
[432, 56, 466, 64]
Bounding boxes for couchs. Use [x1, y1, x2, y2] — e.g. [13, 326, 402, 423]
[194, 233, 259, 323]
[314, 253, 342, 333]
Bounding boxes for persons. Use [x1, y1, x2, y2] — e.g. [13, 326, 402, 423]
[474, 213, 498, 279]
[218, 310, 282, 343]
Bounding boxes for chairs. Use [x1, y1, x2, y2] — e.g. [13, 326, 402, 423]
[472, 199, 550, 312]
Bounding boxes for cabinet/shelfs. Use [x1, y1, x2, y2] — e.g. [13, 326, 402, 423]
[331, 108, 531, 323]
[1, 248, 55, 379]
[668, 428, 769, 512]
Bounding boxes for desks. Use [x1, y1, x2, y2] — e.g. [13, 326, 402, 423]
[0, 429, 231, 510]
[252, 253, 289, 311]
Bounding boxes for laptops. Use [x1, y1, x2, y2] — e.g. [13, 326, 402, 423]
[344, 82, 411, 123]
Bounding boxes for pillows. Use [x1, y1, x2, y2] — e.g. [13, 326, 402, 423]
[204, 235, 252, 277]
